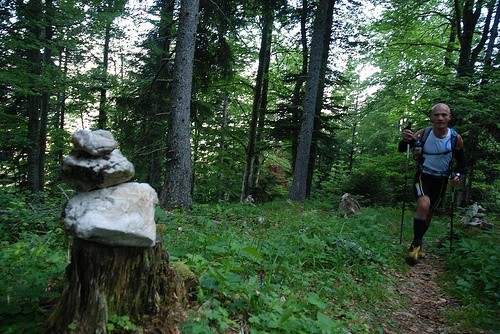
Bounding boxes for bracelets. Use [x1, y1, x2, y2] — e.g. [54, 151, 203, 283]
[456, 172, 462, 178]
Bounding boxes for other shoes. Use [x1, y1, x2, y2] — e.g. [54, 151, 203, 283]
[418, 246, 425, 257]
[408, 245, 420, 262]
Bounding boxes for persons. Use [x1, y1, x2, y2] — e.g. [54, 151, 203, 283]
[397, 102, 466, 265]
